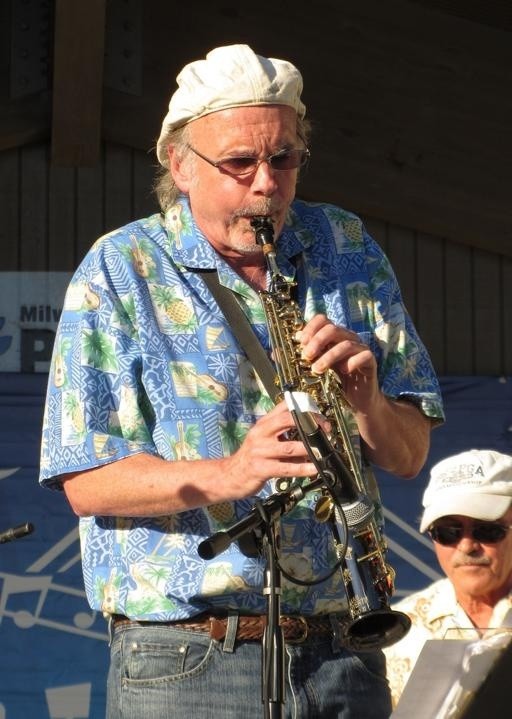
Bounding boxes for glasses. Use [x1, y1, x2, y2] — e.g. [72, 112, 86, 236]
[186, 132, 312, 175]
[427, 521, 509, 545]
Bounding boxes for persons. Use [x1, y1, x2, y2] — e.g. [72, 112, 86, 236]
[39, 43, 448, 718]
[381, 448, 511, 719]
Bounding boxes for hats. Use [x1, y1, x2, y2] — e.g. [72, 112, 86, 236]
[418, 448, 512, 533]
[154, 44, 308, 169]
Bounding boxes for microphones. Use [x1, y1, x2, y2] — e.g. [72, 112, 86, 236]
[284, 390, 375, 531]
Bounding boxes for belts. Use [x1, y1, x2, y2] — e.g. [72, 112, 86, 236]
[178, 616, 353, 642]
